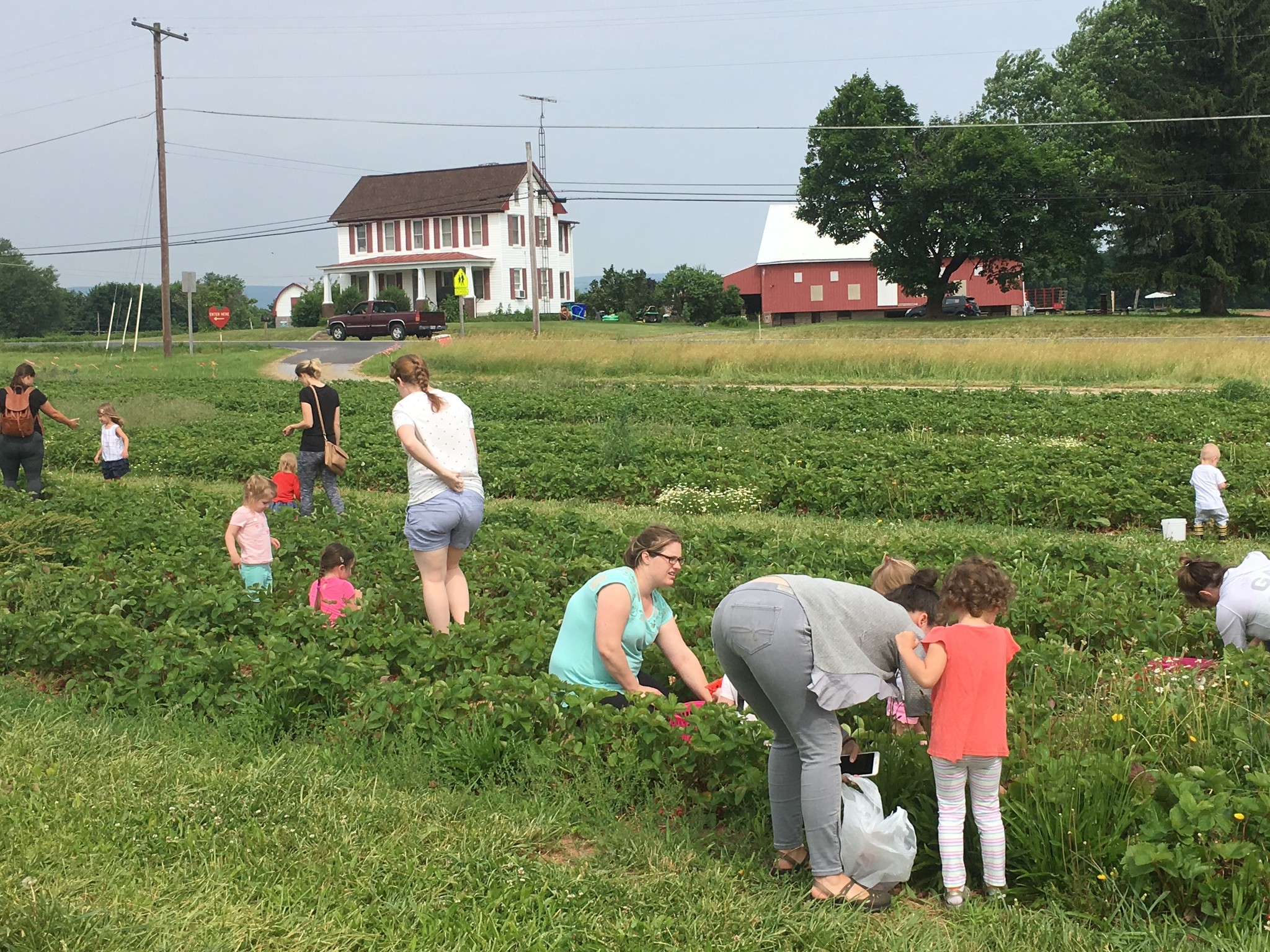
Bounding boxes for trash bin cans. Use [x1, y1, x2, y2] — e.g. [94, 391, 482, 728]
[561, 302, 587, 320]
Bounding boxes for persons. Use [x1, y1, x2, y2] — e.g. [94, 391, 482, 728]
[310, 541, 362, 627]
[360, 308, 367, 314]
[718, 672, 770, 749]
[94, 403, 129, 482]
[225, 474, 280, 603]
[283, 358, 344, 524]
[0, 363, 80, 499]
[896, 559, 1021, 906]
[710, 569, 941, 911]
[1189, 443, 1231, 543]
[270, 453, 300, 522]
[388, 355, 484, 634]
[1177, 550, 1270, 653]
[550, 526, 715, 714]
[872, 553, 929, 746]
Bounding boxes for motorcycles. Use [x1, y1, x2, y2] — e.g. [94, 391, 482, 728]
[633, 305, 662, 323]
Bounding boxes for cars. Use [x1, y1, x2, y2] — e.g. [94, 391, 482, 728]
[1026, 301, 1037, 315]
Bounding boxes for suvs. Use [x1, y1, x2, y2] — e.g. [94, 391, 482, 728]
[904, 293, 981, 318]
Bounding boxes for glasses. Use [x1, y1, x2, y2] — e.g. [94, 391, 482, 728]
[649, 550, 686, 565]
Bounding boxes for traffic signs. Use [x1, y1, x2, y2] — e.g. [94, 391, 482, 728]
[208, 304, 231, 328]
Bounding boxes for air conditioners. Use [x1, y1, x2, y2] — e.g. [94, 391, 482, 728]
[515, 290, 526, 298]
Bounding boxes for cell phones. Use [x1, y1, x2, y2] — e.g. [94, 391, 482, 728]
[839, 751, 880, 776]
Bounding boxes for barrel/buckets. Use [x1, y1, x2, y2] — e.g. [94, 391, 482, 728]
[1160, 518, 1189, 542]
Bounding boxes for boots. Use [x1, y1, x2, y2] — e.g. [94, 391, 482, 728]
[1192, 524, 1204, 540]
[1217, 524, 1227, 541]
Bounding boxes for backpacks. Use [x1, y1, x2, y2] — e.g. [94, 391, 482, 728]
[2, 387, 37, 436]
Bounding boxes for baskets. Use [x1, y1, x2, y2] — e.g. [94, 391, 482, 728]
[1133, 656, 1213, 693]
[705, 676, 723, 701]
[671, 702, 708, 740]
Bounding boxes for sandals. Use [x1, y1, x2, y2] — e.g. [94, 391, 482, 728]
[982, 884, 1008, 897]
[942, 885, 971, 908]
[809, 876, 891, 910]
[771, 849, 812, 876]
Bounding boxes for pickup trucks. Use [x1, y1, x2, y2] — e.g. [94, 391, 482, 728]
[326, 297, 448, 341]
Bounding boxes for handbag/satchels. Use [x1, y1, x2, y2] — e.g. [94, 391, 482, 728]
[840, 773, 917, 891]
[325, 441, 349, 477]
